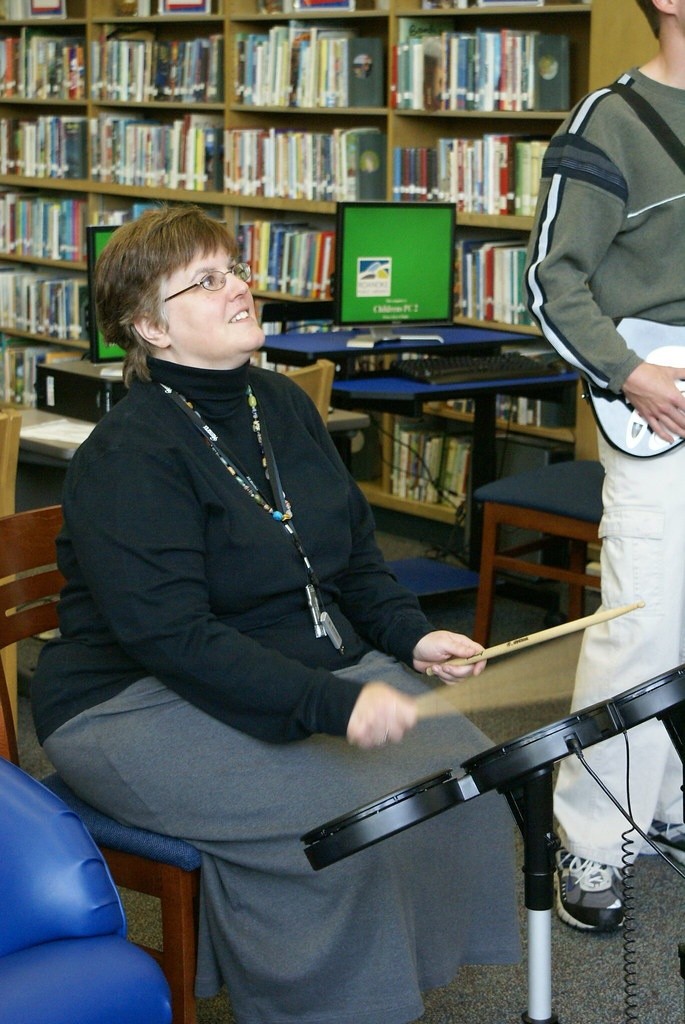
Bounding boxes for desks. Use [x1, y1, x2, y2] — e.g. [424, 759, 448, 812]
[259, 326, 582, 628]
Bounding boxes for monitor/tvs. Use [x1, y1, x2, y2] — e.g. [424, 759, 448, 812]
[333, 200, 457, 349]
[86, 225, 129, 363]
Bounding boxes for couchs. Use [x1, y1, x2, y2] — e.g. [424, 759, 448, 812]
[0, 756, 172, 1024]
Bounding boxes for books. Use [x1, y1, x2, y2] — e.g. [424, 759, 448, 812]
[2, 0, 575, 510]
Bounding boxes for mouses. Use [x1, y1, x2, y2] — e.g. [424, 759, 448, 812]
[533, 352, 572, 374]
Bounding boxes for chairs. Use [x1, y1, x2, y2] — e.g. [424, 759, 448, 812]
[0, 502, 204, 1024]
[473, 458, 606, 645]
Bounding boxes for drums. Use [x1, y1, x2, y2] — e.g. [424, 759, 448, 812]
[300, 658, 685, 872]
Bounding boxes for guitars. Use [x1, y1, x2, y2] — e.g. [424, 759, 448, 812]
[579, 314, 685, 458]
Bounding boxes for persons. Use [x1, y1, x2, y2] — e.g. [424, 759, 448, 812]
[524, 1, 684, 929]
[32, 204, 531, 1023]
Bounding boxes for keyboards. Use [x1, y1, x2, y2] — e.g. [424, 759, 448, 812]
[390, 350, 560, 386]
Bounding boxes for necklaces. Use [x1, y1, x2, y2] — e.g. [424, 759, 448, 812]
[160, 382, 294, 522]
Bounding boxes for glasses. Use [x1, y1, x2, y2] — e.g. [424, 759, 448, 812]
[162, 262, 252, 302]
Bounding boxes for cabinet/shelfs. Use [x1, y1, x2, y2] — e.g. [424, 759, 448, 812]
[0, 0, 659, 562]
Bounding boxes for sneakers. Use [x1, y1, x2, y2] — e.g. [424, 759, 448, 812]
[553, 844, 627, 931]
[638, 819, 685, 867]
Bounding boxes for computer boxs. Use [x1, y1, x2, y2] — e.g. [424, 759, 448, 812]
[462, 429, 576, 584]
